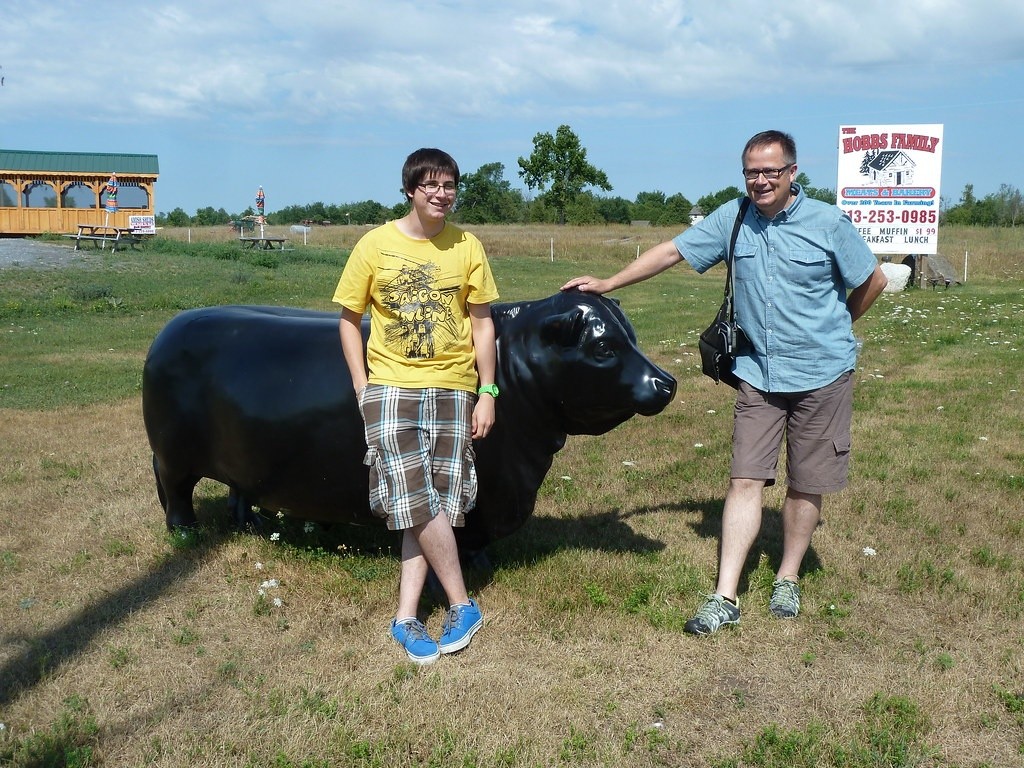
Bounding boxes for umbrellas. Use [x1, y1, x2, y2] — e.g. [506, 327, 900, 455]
[256, 186, 265, 238]
[102, 172, 118, 251]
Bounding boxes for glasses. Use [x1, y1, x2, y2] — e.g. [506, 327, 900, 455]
[417, 183, 457, 193]
[742, 162, 795, 180]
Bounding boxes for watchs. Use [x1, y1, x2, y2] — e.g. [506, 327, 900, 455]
[478, 384, 499, 397]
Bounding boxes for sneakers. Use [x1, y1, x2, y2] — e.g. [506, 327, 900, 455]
[684, 594, 741, 635]
[391, 616, 440, 665]
[769, 575, 802, 620]
[438, 599, 481, 654]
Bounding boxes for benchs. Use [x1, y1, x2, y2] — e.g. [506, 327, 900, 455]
[62, 235, 146, 245]
[248, 248, 294, 252]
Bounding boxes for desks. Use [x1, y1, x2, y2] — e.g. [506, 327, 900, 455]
[239, 237, 290, 250]
[74, 224, 141, 253]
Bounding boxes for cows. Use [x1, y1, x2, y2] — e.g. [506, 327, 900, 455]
[143, 286, 679, 611]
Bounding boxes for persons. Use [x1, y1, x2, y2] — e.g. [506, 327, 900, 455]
[561, 131, 888, 636]
[902, 254, 918, 288]
[332, 148, 500, 666]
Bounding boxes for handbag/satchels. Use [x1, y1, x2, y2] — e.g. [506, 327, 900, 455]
[698, 305, 751, 390]
